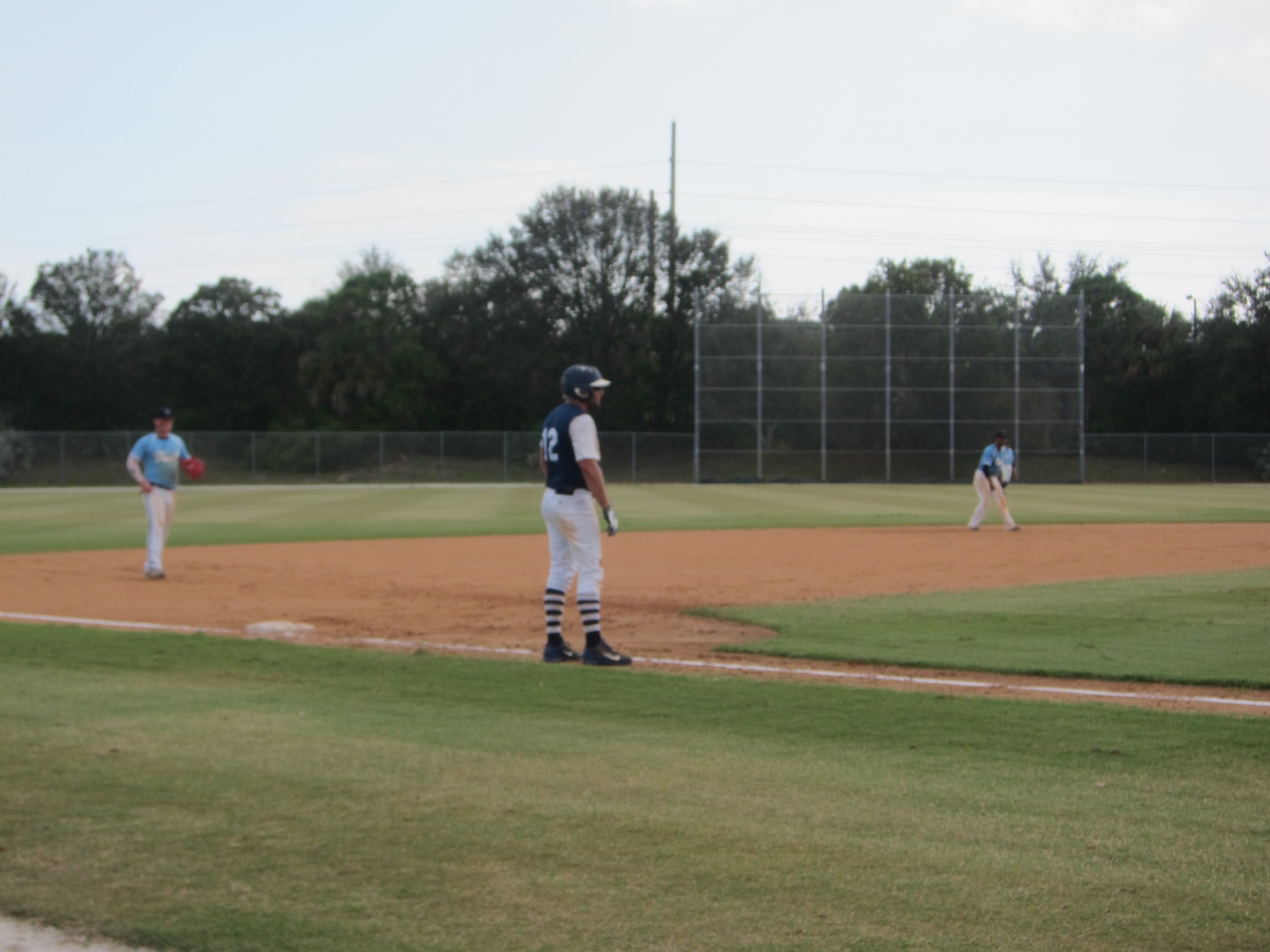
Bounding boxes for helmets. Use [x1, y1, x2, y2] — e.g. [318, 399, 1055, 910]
[560, 364, 611, 408]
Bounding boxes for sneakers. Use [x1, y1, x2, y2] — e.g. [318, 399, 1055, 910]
[582, 639, 632, 666]
[542, 641, 582, 663]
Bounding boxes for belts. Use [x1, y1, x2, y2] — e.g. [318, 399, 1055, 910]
[150, 483, 172, 490]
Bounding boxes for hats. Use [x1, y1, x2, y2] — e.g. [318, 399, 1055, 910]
[152, 405, 175, 419]
[994, 431, 1008, 440]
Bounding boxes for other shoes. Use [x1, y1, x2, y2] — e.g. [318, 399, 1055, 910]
[144, 568, 165, 580]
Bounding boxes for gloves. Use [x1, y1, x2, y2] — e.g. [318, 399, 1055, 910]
[139, 479, 152, 492]
[603, 507, 618, 536]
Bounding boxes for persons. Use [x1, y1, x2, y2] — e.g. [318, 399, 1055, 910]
[539, 364, 632, 665]
[126, 405, 204, 580]
[967, 429, 1020, 530]
